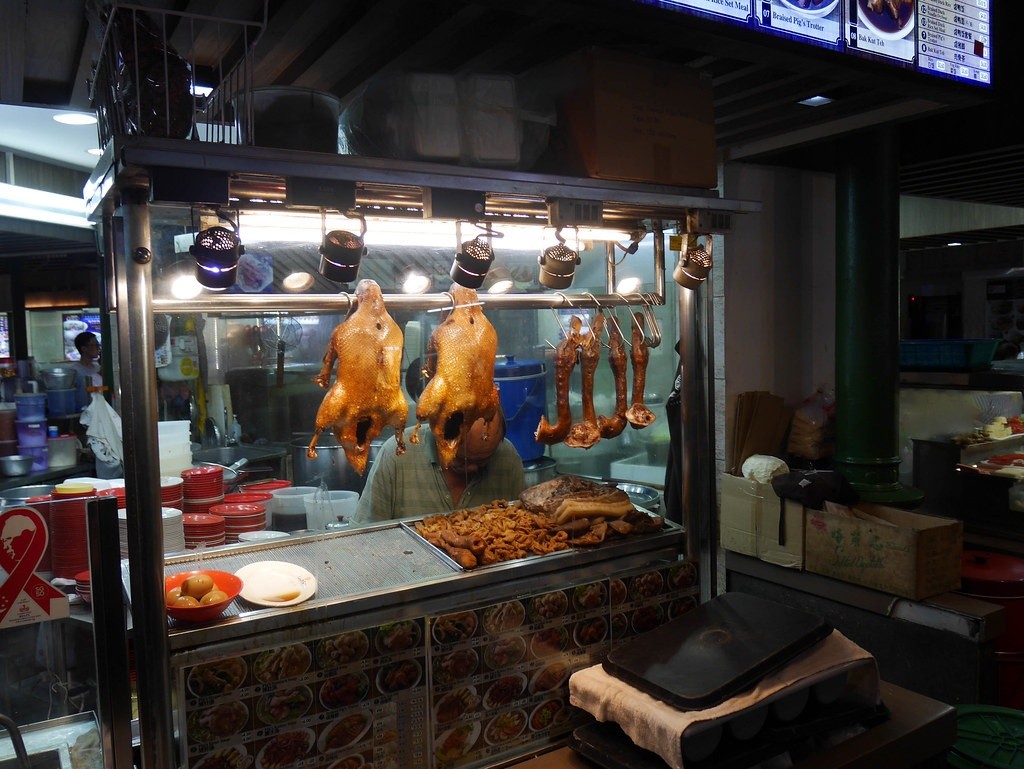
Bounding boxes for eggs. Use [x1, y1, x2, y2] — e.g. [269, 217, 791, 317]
[173, 596, 200, 606]
[200, 591, 229, 605]
[181, 575, 213, 598]
[167, 587, 181, 606]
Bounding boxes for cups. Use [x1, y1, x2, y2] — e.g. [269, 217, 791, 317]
[49, 426, 58, 438]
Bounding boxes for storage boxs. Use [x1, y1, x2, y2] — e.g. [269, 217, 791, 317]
[900, 338, 1003, 391]
[804, 501, 964, 601]
[720, 472, 781, 557]
[516, 45, 720, 190]
[784, 500, 845, 560]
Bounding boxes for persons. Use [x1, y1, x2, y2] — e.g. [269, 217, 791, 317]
[348, 398, 526, 528]
[69, 331, 103, 374]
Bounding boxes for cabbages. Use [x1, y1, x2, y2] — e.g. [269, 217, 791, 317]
[741, 454, 789, 483]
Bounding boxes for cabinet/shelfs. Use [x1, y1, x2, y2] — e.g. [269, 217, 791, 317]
[0, 464, 94, 493]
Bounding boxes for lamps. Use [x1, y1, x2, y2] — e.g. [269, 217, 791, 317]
[317, 208, 367, 281]
[672, 209, 714, 291]
[536, 225, 581, 289]
[189, 202, 246, 291]
[449, 222, 504, 289]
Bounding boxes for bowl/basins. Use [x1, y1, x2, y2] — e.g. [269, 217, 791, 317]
[573, 583, 607, 614]
[0, 455, 34, 476]
[612, 612, 628, 639]
[166, 569, 243, 622]
[667, 595, 697, 621]
[611, 578, 627, 606]
[50, 480, 97, 603]
[632, 604, 664, 634]
[667, 563, 697, 592]
[39, 367, 77, 389]
[629, 571, 664, 601]
[572, 616, 608, 647]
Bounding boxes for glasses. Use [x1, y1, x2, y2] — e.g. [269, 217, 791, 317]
[84, 343, 100, 348]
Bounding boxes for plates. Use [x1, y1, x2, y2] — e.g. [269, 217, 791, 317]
[484, 708, 528, 746]
[529, 660, 571, 698]
[374, 620, 421, 658]
[434, 686, 477, 725]
[433, 648, 479, 684]
[483, 600, 526, 635]
[376, 660, 422, 696]
[316, 631, 368, 670]
[529, 697, 564, 733]
[527, 590, 568, 623]
[62, 466, 292, 556]
[317, 709, 372, 754]
[187, 656, 248, 699]
[432, 610, 478, 645]
[256, 684, 313, 726]
[435, 720, 481, 762]
[233, 560, 317, 607]
[482, 672, 528, 712]
[484, 633, 526, 670]
[319, 672, 370, 712]
[187, 699, 249, 744]
[256, 728, 316, 769]
[253, 642, 312, 686]
[530, 626, 569, 660]
[192, 742, 248, 769]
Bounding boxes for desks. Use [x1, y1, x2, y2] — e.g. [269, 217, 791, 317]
[47, 413, 81, 433]
[906, 427, 1024, 531]
[504, 676, 958, 769]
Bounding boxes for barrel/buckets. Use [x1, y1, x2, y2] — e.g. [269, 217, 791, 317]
[270, 486, 322, 532]
[304, 490, 360, 530]
[47, 434, 78, 467]
[494, 353, 546, 463]
[0, 485, 55, 512]
